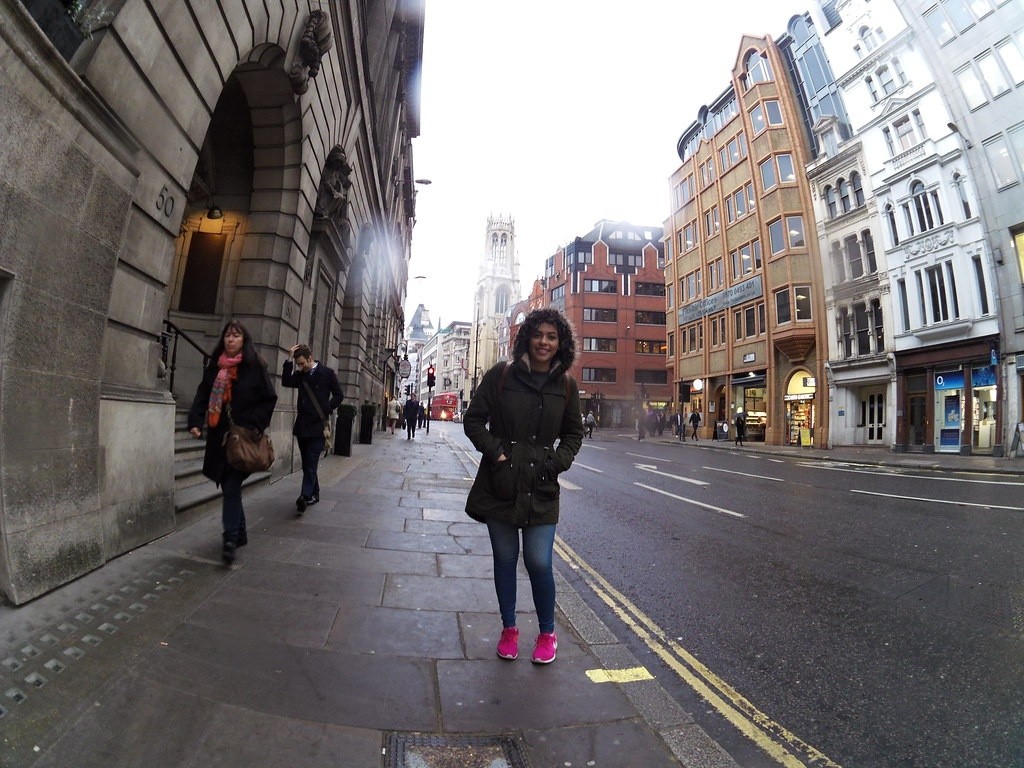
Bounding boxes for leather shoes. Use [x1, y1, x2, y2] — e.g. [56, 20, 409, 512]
[223, 537, 248, 562]
[296, 493, 319, 513]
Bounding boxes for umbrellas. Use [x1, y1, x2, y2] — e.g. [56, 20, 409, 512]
[735, 412, 748, 417]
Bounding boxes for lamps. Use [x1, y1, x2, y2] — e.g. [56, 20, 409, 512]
[394, 178, 432, 185]
[208, 194, 222, 219]
[948, 123, 973, 149]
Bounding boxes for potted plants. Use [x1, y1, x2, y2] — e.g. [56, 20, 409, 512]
[335, 404, 357, 457]
[359, 405, 376, 444]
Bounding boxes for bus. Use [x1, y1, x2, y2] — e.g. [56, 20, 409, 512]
[430, 393, 456, 421]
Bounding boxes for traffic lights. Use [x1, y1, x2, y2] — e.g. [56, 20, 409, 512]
[427, 365, 435, 388]
[591, 392, 606, 407]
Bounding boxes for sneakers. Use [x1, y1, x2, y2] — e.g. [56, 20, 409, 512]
[495, 626, 520, 660]
[531, 632, 558, 664]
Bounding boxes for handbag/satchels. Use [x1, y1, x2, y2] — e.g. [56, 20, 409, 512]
[402, 421, 407, 430]
[744, 428, 750, 437]
[323, 414, 332, 451]
[225, 425, 276, 473]
[396, 405, 400, 413]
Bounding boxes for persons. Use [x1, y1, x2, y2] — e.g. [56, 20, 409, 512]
[464, 307, 587, 663]
[585, 411, 597, 438]
[735, 417, 746, 446]
[405, 393, 419, 440]
[674, 410, 681, 437]
[387, 397, 400, 434]
[188, 319, 278, 561]
[689, 409, 701, 441]
[649, 411, 665, 435]
[419, 403, 424, 428]
[281, 344, 344, 513]
